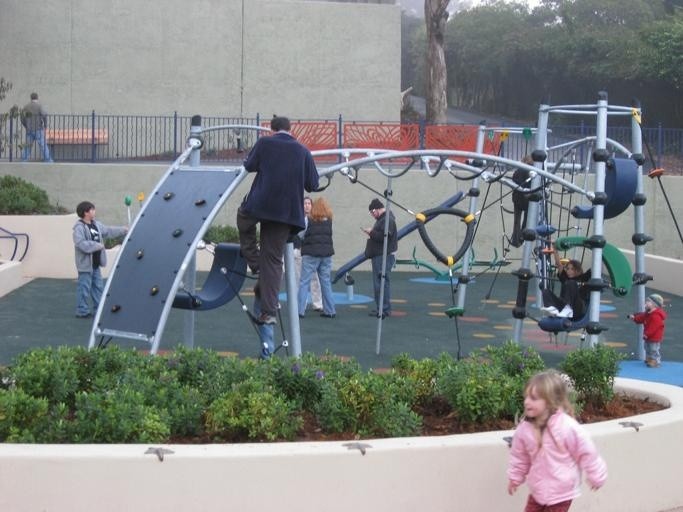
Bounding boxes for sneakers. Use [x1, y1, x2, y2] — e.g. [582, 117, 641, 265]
[257, 313, 277, 325]
[645, 358, 659, 369]
[368, 310, 390, 316]
[540, 305, 573, 318]
[298, 308, 336, 318]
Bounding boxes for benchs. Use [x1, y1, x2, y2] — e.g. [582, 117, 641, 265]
[43, 129, 109, 160]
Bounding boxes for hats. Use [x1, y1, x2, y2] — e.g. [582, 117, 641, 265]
[649, 294, 664, 307]
[369, 199, 383, 209]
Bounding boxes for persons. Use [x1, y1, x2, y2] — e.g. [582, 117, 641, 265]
[237, 117, 318, 326]
[506, 369, 608, 512]
[72, 201, 129, 318]
[360, 198, 397, 317]
[628, 293, 666, 367]
[297, 197, 335, 319]
[540, 260, 589, 322]
[19, 94, 54, 163]
[512, 154, 534, 248]
